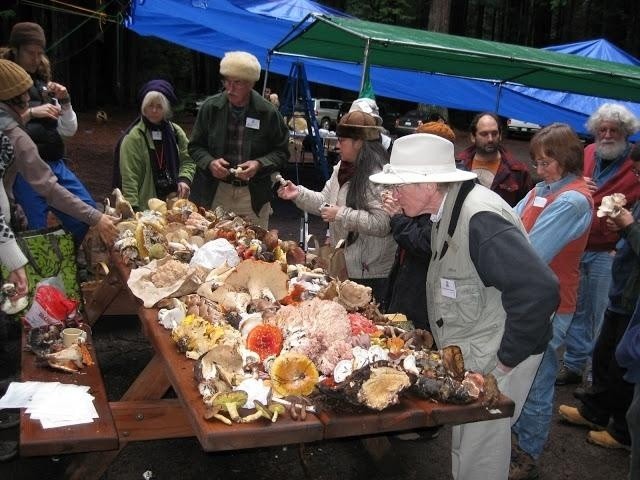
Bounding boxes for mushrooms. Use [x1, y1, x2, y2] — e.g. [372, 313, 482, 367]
[1, 283, 29, 315]
[104, 167, 500, 426]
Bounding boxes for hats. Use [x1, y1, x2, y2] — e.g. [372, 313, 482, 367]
[369, 133, 477, 184]
[140, 80, 177, 103]
[220, 51, 261, 80]
[350, 97, 382, 125]
[417, 122, 456, 142]
[337, 111, 381, 141]
[0, 59, 34, 101]
[10, 21, 45, 46]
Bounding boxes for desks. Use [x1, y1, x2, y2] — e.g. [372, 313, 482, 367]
[68, 198, 516, 479]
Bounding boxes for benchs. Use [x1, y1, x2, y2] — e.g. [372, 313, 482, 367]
[20, 230, 130, 479]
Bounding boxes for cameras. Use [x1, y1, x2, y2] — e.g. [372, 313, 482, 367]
[153, 170, 178, 198]
[41, 86, 57, 97]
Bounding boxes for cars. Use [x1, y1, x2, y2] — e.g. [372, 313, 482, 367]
[507, 118, 545, 140]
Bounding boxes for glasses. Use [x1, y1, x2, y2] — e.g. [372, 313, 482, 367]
[531, 159, 557, 168]
[223, 82, 249, 87]
[390, 184, 405, 192]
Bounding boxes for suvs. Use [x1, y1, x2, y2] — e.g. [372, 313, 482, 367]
[297, 96, 456, 143]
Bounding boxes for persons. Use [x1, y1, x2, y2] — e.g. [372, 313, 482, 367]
[0, 57, 120, 249]
[347, 96, 640, 480]
[186, 48, 291, 231]
[273, 109, 404, 301]
[112, 79, 197, 211]
[0, 21, 98, 245]
[1, 131, 31, 315]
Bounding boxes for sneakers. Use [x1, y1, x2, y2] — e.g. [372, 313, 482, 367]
[556, 367, 582, 385]
[559, 406, 606, 429]
[588, 431, 631, 451]
[508, 445, 538, 479]
[573, 386, 595, 399]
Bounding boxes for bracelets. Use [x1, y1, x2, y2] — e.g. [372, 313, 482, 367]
[58, 96, 72, 105]
[28, 106, 34, 119]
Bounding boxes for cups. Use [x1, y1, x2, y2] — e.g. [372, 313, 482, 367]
[62, 328, 83, 348]
